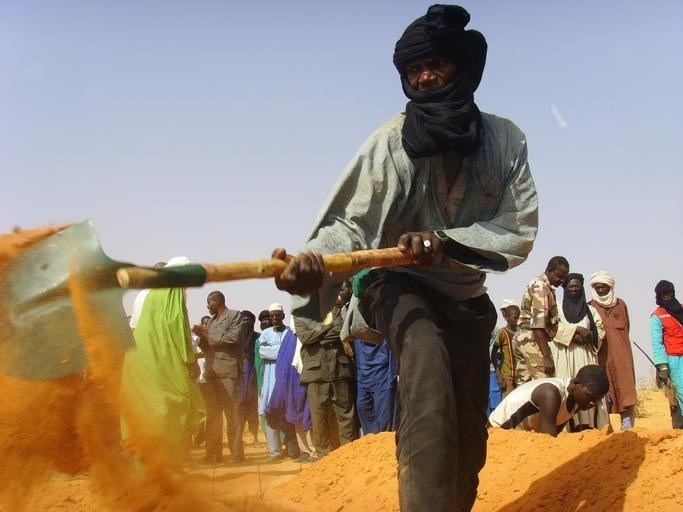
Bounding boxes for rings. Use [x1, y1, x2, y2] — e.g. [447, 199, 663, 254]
[423, 240, 432, 250]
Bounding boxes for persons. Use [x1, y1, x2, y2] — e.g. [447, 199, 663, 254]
[271, 4, 539, 511]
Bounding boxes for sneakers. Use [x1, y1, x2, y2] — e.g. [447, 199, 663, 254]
[199, 452, 326, 466]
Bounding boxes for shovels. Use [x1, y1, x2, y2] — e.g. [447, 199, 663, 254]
[0, 218, 422, 379]
